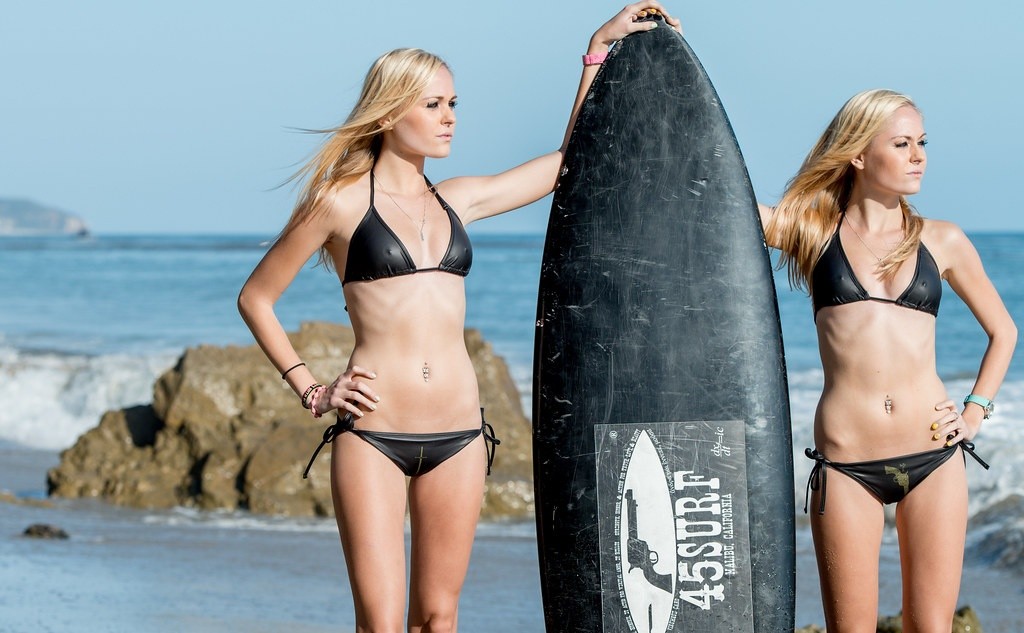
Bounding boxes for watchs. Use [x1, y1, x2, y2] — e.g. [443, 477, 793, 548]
[963, 396, 995, 419]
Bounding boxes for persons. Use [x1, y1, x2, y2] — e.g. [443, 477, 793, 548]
[239, 0, 668, 633]
[633, 9, 1017, 633]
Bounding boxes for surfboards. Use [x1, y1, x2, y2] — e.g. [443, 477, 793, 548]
[533, 17, 797, 633]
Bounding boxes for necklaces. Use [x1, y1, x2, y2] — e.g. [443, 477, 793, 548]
[843, 209, 903, 262]
[374, 168, 430, 240]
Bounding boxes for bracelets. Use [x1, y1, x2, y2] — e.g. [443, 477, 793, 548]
[302, 383, 326, 418]
[583, 54, 610, 65]
[282, 363, 305, 379]
